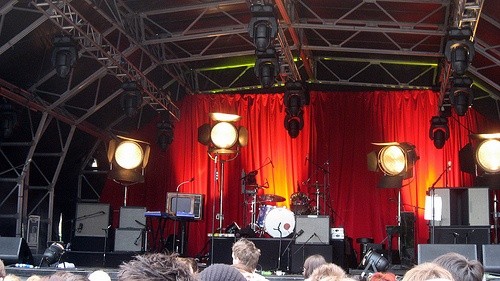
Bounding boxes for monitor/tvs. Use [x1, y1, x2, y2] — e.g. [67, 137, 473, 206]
[166, 192, 202, 219]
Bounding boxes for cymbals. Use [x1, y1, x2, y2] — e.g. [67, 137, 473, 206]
[257, 193, 286, 202]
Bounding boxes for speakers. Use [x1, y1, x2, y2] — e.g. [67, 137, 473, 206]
[417, 243, 500, 273]
[211, 236, 333, 274]
[0, 237, 35, 266]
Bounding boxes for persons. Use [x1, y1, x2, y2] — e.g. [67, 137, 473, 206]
[232, 239, 271, 281]
[0, 254, 484, 281]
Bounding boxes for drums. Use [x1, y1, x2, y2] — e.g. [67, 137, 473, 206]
[256, 204, 275, 228]
[263, 207, 296, 238]
